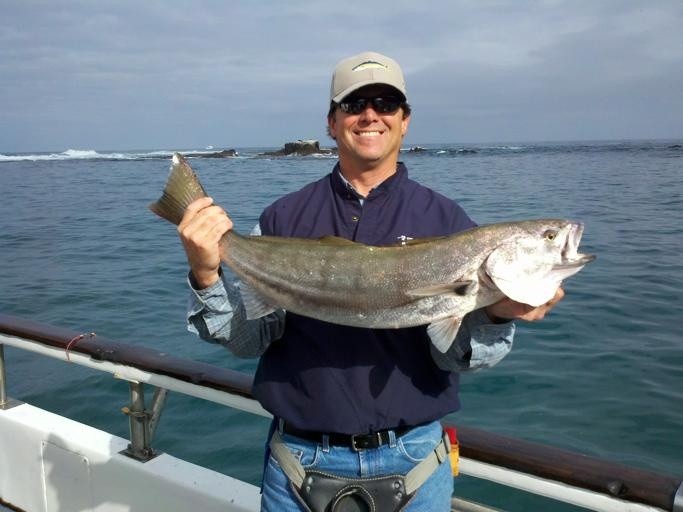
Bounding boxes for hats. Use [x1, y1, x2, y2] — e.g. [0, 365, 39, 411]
[329, 51, 407, 106]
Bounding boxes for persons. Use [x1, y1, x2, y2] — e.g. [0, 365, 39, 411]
[177, 52, 564, 512]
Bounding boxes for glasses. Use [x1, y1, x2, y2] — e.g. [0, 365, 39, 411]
[339, 91, 401, 115]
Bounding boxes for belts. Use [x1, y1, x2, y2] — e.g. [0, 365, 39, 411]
[276, 416, 431, 452]
